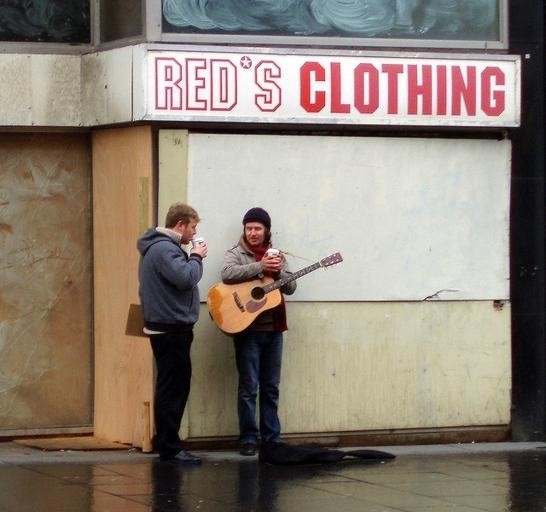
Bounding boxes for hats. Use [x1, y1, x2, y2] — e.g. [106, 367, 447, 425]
[243, 207, 271, 228]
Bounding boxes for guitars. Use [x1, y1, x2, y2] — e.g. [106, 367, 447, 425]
[206, 251, 343, 337]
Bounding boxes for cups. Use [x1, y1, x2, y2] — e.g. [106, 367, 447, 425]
[192, 238, 206, 258]
[267, 249, 280, 257]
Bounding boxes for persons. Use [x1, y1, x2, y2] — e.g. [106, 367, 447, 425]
[222, 206, 296, 455]
[135, 202, 208, 465]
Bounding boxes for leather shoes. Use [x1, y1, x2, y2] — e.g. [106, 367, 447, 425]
[240, 443, 256, 455]
[159, 448, 201, 464]
